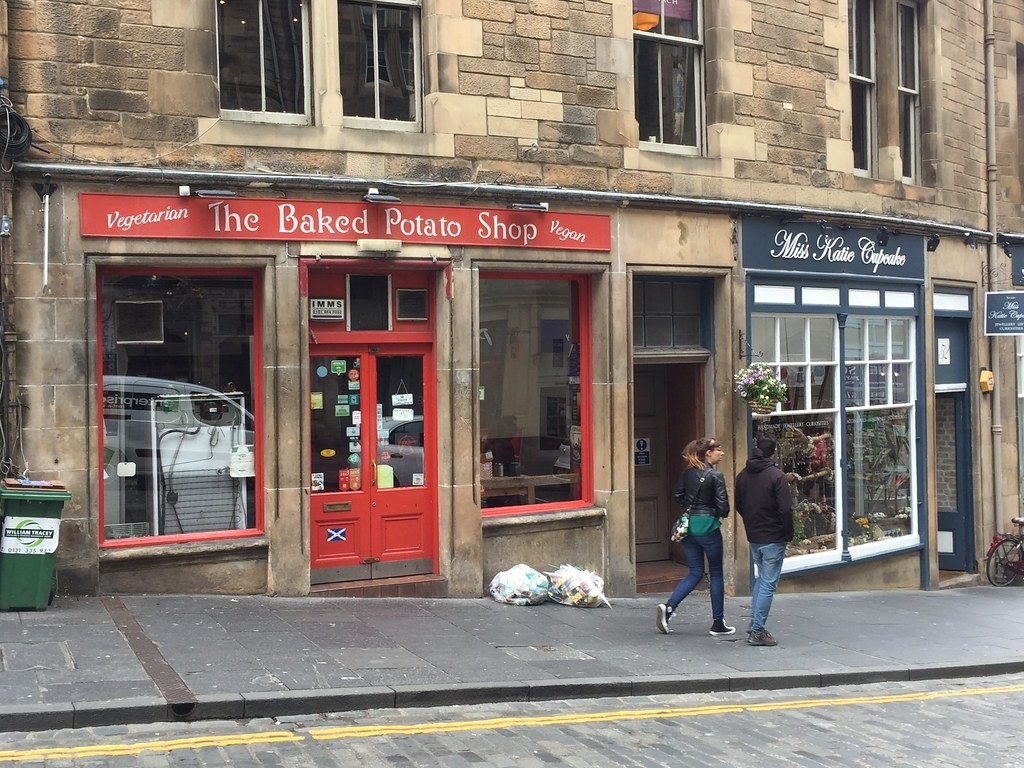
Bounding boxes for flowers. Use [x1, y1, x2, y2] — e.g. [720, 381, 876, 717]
[732, 360, 788, 407]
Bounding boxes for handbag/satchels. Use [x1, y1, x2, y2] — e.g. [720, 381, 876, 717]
[671, 508, 690, 542]
[490, 562, 549, 606]
[547, 564, 605, 609]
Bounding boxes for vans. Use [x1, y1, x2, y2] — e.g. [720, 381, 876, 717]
[101, 374, 255, 490]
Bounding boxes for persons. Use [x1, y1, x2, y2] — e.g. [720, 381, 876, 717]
[496, 422, 520, 505]
[734, 436, 794, 647]
[484, 414, 517, 509]
[655, 436, 736, 637]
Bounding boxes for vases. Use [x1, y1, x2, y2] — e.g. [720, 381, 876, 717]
[747, 396, 778, 414]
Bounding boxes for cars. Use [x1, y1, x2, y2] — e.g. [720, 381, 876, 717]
[376, 414, 425, 487]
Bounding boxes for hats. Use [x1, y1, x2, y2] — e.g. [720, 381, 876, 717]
[758, 438, 776, 456]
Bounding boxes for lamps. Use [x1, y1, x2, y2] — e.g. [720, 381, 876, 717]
[978, 239, 1012, 258]
[840, 223, 889, 247]
[507, 203, 546, 211]
[780, 219, 835, 240]
[940, 232, 977, 250]
[364, 194, 402, 205]
[892, 229, 941, 252]
[195, 187, 237, 201]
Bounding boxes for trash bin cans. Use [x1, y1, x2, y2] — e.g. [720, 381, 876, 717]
[0, 478, 72, 609]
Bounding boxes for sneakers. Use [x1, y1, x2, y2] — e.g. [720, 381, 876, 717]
[709, 618, 736, 635]
[656, 604, 676, 634]
[745, 625, 777, 646]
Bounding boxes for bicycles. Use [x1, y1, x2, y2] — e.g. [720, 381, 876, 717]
[982, 517, 1024, 587]
